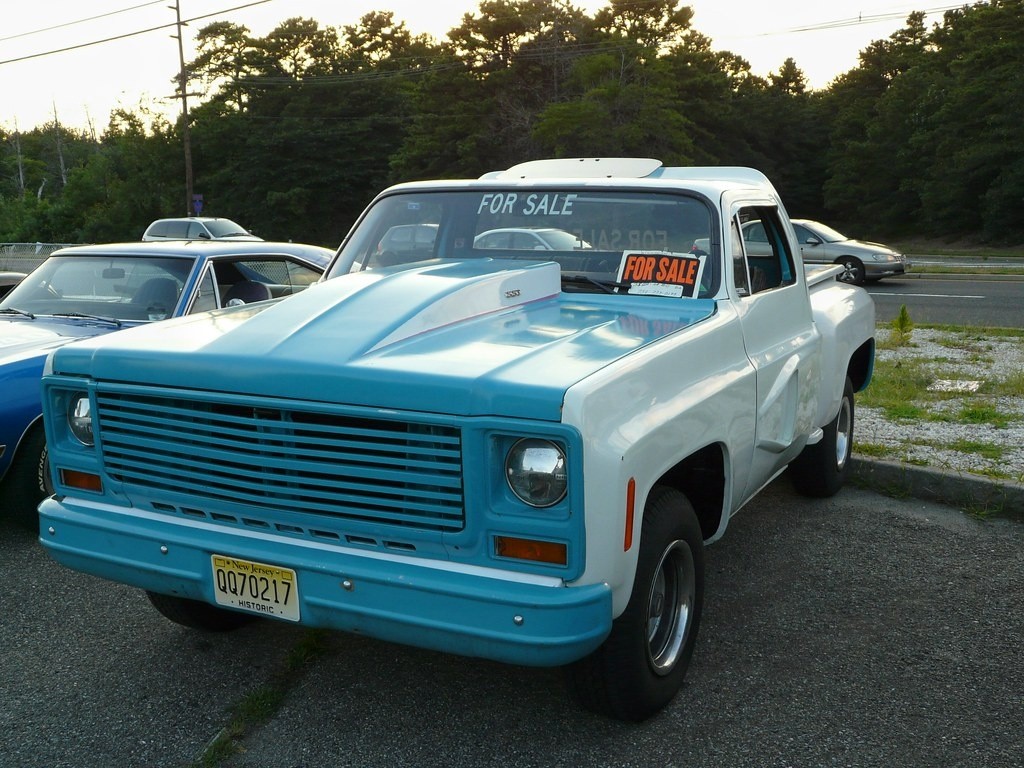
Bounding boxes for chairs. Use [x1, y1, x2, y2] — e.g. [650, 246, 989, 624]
[733, 265, 766, 297]
[129, 277, 181, 307]
[220, 280, 272, 309]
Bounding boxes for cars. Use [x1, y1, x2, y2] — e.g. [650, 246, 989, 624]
[0, 240, 373, 508]
[472, 227, 610, 255]
[689, 218, 907, 288]
[36, 155, 878, 709]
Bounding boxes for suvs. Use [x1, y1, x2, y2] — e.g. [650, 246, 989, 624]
[141, 217, 266, 244]
[376, 224, 441, 266]
